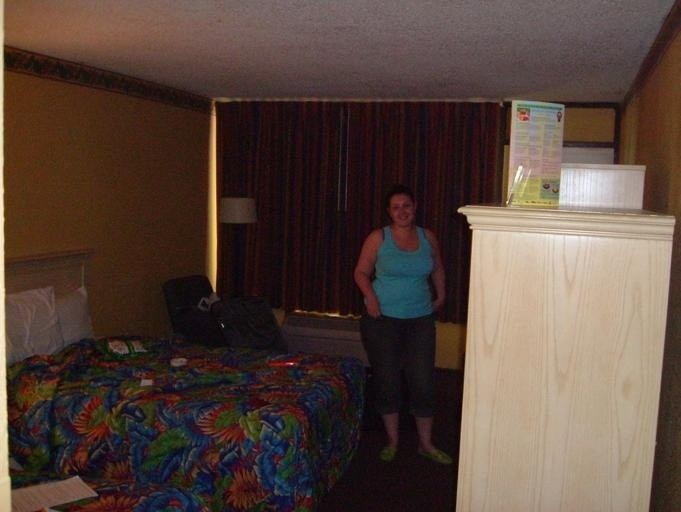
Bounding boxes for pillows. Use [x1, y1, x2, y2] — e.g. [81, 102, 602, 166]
[4, 256, 213, 367]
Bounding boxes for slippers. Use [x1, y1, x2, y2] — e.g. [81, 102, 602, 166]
[418, 447, 452, 464]
[379, 446, 397, 461]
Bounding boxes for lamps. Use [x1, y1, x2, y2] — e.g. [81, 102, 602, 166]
[220, 198, 258, 298]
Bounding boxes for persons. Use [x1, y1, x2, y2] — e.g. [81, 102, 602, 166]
[353, 185, 454, 466]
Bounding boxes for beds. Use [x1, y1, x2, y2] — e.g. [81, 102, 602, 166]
[4, 249, 363, 512]
